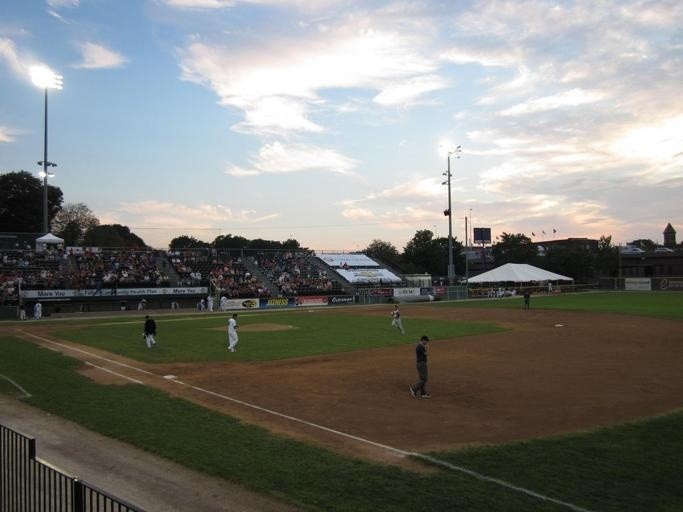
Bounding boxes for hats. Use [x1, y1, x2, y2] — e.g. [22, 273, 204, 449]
[420, 335, 430, 342]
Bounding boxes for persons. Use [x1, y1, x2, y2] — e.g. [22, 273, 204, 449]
[224, 312, 241, 353]
[408, 335, 431, 399]
[378, 277, 384, 288]
[388, 303, 406, 335]
[487, 279, 553, 299]
[0, 238, 342, 323]
[141, 315, 158, 349]
[343, 261, 348, 270]
[523, 290, 530, 311]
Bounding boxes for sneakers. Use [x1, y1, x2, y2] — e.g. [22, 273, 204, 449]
[409, 384, 431, 399]
